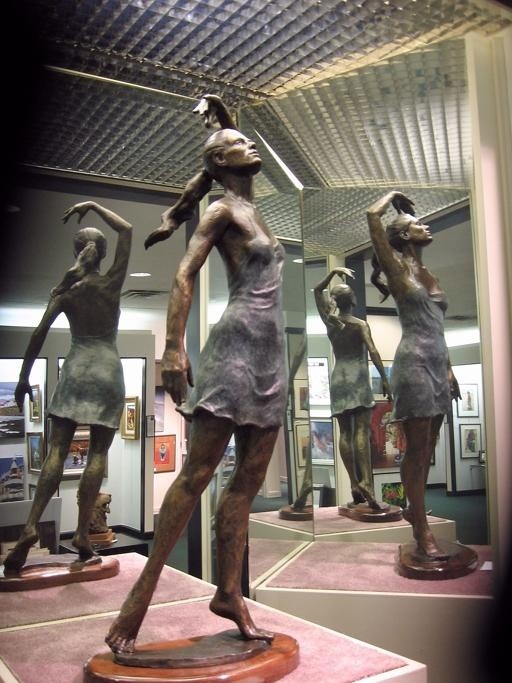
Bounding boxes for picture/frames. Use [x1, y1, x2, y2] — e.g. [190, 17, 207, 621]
[26, 384, 46, 495]
[362, 356, 484, 514]
[153, 434, 177, 473]
[120, 397, 139, 441]
[283, 326, 337, 507]
[60, 434, 108, 481]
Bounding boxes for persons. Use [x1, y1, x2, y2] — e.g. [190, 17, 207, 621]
[362, 186, 465, 563]
[4, 199, 135, 573]
[99, 90, 293, 655]
[311, 265, 390, 511]
[288, 330, 312, 510]
